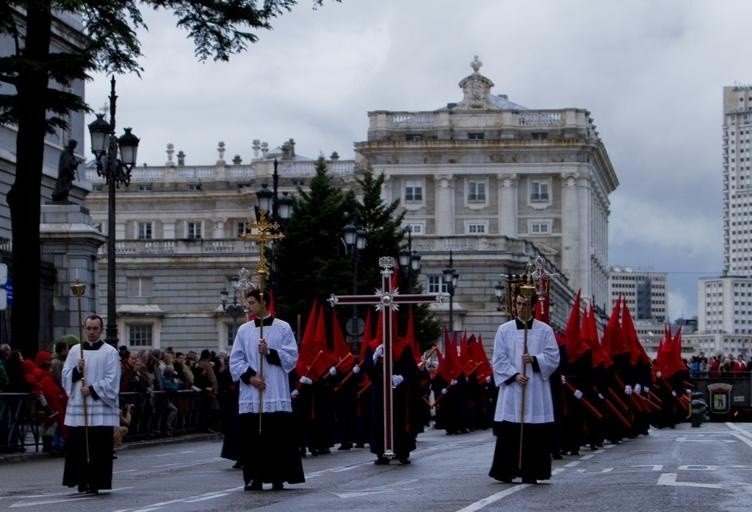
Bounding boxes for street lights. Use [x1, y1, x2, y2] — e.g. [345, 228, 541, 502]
[441, 248, 460, 334]
[397, 229, 422, 314]
[88, 72, 139, 348]
[340, 216, 369, 354]
[495, 272, 534, 320]
[253, 158, 295, 312]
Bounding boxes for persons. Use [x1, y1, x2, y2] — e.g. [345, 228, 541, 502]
[61, 314, 122, 494]
[1, 342, 71, 455]
[548, 334, 752, 465]
[228, 288, 306, 491]
[285, 333, 499, 464]
[49, 137, 85, 202]
[488, 290, 560, 483]
[116, 344, 236, 441]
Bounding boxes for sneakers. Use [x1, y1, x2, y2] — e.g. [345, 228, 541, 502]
[78, 485, 98, 495]
[487, 414, 710, 485]
[232, 419, 492, 489]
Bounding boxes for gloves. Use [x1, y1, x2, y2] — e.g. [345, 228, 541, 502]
[562, 369, 692, 400]
[291, 343, 494, 399]
